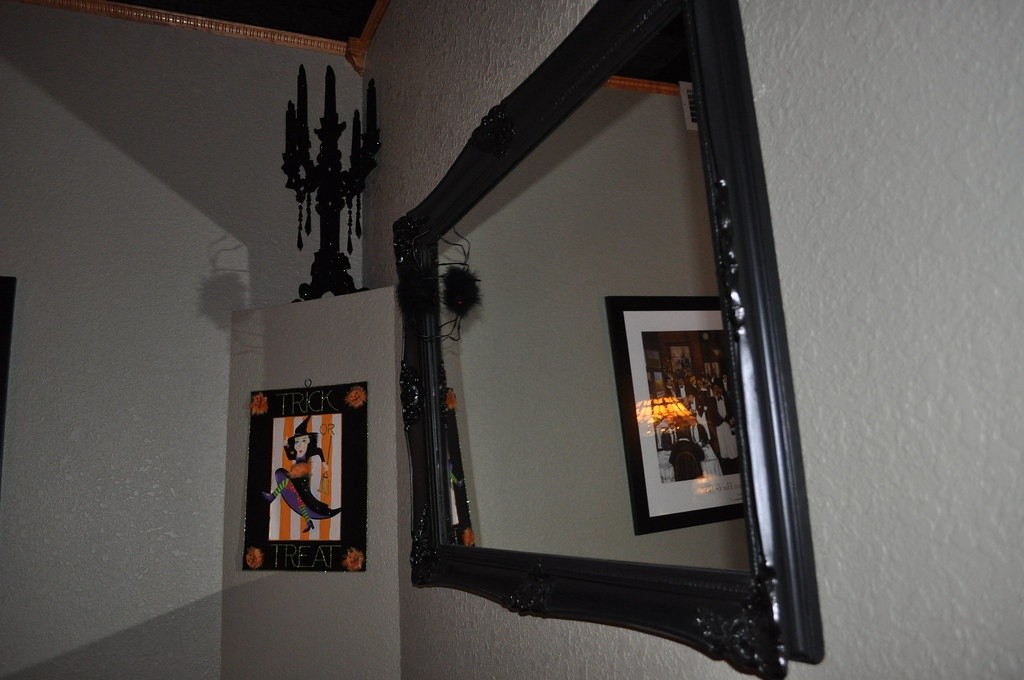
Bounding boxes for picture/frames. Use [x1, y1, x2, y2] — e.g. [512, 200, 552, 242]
[604, 296, 744, 536]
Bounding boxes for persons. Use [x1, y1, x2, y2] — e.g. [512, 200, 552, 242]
[661, 376, 742, 482]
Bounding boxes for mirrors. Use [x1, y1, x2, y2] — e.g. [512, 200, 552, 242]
[394, 1, 824, 680]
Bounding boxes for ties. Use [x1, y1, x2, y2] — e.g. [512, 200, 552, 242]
[672, 431, 676, 444]
[692, 426, 696, 442]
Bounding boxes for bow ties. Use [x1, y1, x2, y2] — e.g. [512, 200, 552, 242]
[718, 397, 722, 402]
[690, 401, 693, 404]
[680, 386, 684, 389]
